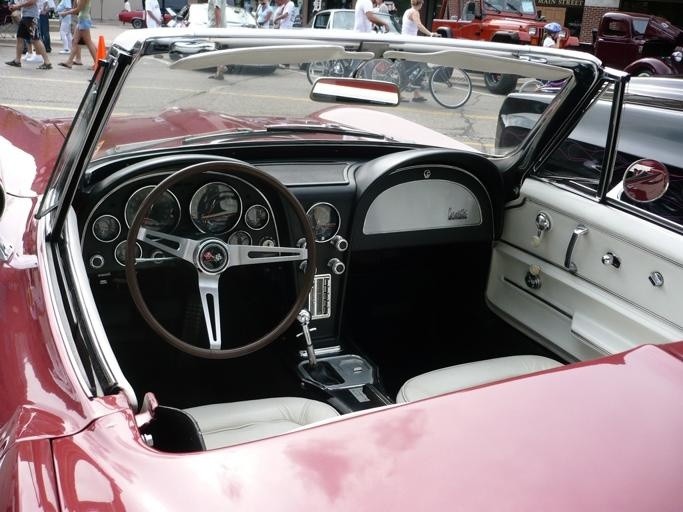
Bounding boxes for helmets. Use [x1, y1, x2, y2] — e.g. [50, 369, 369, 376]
[544, 23, 561, 32]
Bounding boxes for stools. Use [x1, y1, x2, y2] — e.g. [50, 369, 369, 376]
[178, 395, 343, 453]
[393, 354, 564, 406]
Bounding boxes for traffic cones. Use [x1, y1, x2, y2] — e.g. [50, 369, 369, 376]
[87, 35, 109, 85]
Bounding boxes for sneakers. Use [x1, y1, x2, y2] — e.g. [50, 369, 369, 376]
[5, 60, 21, 67]
[37, 63, 53, 69]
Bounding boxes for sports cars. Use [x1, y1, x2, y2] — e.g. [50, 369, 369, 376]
[0, 26, 683, 512]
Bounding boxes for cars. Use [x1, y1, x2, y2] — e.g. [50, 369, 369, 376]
[493, 68, 680, 224]
[115, 0, 683, 94]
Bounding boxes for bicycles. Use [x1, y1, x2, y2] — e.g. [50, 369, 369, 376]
[353, 33, 475, 110]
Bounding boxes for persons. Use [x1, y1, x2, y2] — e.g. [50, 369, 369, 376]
[207, 0, 228, 80]
[122, 0, 132, 27]
[144, 0, 164, 58]
[543, 22, 562, 49]
[4, 0, 98, 72]
[255, 0, 296, 69]
[351, 0, 437, 103]
